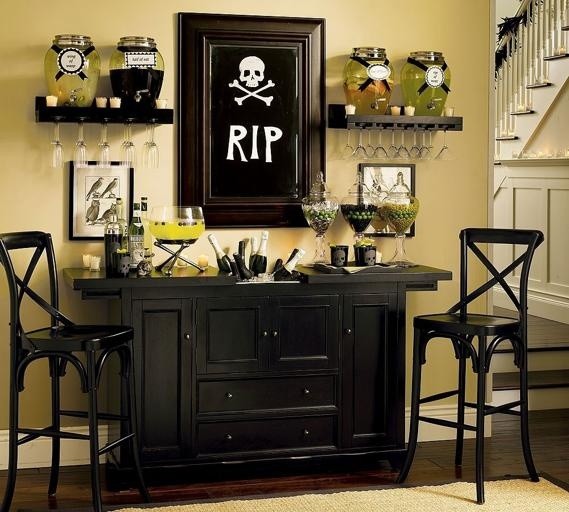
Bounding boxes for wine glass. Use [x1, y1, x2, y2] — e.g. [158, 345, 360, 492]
[119, 117, 136, 168]
[339, 128, 456, 162]
[72, 117, 89, 169]
[142, 118, 159, 170]
[45, 116, 66, 169]
[95, 118, 111, 169]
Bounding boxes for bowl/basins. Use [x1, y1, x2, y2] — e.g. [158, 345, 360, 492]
[147, 205, 206, 244]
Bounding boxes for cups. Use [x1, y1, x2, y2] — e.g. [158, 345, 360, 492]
[176, 254, 188, 268]
[197, 255, 209, 268]
[443, 105, 454, 117]
[330, 242, 377, 267]
[156, 98, 168, 109]
[80, 253, 102, 272]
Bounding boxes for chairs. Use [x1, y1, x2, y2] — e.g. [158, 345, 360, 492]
[396, 228, 546, 504]
[0, 232, 150, 511]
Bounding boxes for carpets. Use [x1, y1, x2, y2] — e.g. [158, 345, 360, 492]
[109, 469, 568, 512]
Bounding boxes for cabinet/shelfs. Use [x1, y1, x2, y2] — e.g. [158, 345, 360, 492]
[63, 262, 454, 506]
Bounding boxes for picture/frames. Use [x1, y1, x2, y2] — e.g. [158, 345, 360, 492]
[176, 12, 326, 227]
[357, 163, 416, 237]
[67, 160, 134, 241]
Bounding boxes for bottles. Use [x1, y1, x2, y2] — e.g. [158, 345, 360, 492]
[207, 230, 307, 282]
[103, 195, 153, 276]
[43, 33, 165, 108]
[341, 47, 451, 116]
